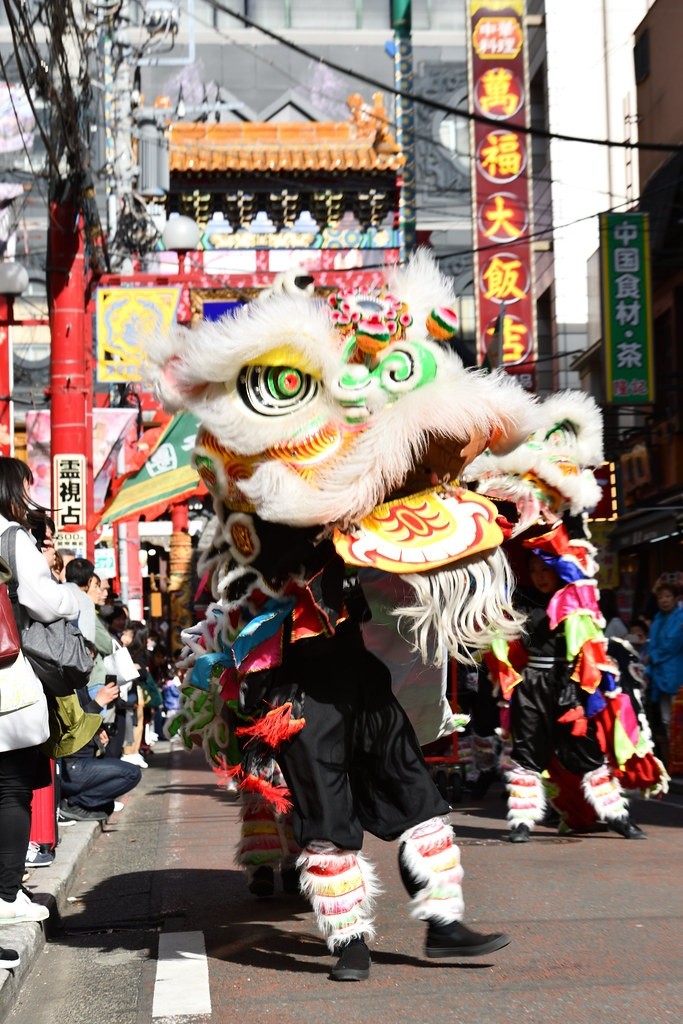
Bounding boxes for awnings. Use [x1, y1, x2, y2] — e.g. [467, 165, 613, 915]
[601, 507, 683, 538]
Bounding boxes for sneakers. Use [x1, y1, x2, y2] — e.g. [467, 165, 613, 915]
[332, 938, 371, 982]
[426, 921, 511, 958]
[0, 889, 50, 925]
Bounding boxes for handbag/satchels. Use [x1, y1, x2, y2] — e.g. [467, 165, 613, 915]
[103, 637, 140, 686]
[0, 583, 21, 664]
[0, 525, 95, 696]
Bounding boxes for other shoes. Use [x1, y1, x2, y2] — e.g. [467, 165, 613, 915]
[0, 947, 21, 968]
[279, 868, 300, 893]
[58, 814, 76, 826]
[605, 817, 648, 839]
[25, 841, 53, 868]
[120, 753, 149, 768]
[511, 822, 530, 842]
[21, 870, 30, 882]
[59, 798, 109, 820]
[246, 864, 273, 897]
[226, 781, 238, 797]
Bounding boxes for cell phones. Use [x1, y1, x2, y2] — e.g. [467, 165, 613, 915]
[26, 509, 47, 553]
[106, 674, 117, 687]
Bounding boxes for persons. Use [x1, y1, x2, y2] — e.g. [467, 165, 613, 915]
[227, 466, 683, 979]
[0, 455, 194, 970]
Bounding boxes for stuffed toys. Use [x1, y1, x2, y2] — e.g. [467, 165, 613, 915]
[143, 250, 672, 801]
